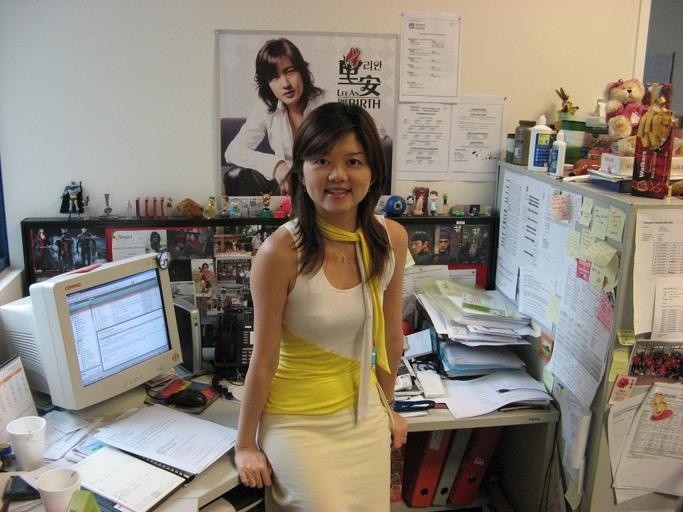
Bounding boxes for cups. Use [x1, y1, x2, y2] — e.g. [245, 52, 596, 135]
[35, 468, 81, 512]
[558, 114, 587, 165]
[6, 415, 47, 471]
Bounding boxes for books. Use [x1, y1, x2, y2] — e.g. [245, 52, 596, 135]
[408, 275, 553, 420]
[66, 401, 239, 511]
[557, 115, 609, 170]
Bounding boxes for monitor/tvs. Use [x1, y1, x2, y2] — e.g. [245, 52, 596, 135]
[0, 253, 184, 417]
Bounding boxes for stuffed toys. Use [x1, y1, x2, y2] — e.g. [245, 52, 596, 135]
[604, 76, 675, 158]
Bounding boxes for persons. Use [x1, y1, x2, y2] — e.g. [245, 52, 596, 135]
[31, 227, 56, 275]
[223, 37, 392, 196]
[196, 262, 213, 297]
[230, 100, 409, 511]
[251, 231, 261, 254]
[55, 226, 75, 272]
[434, 226, 458, 263]
[411, 230, 432, 265]
[57, 180, 85, 222]
[181, 233, 202, 259]
[144, 232, 164, 253]
[175, 231, 191, 251]
[73, 225, 96, 265]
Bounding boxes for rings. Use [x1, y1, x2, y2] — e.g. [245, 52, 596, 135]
[402, 431, 407, 437]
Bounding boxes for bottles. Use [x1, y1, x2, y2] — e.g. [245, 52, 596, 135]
[527, 114, 553, 172]
[512, 119, 536, 166]
[504, 134, 517, 163]
[547, 130, 567, 177]
[630, 81, 679, 198]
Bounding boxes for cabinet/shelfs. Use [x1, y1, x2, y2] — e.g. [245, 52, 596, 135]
[493, 161, 683, 511]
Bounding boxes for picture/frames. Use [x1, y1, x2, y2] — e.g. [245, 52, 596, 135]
[212, 29, 401, 204]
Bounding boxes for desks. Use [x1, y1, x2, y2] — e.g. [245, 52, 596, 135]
[0, 268, 562, 511]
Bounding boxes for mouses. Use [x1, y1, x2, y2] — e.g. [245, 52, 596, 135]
[165, 388, 208, 408]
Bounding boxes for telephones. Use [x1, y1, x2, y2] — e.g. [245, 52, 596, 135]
[215, 313, 254, 377]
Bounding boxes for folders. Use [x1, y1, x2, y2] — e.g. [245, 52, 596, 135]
[402, 427, 498, 507]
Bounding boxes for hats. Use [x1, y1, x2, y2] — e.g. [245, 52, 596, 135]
[412, 230, 430, 241]
[440, 227, 450, 240]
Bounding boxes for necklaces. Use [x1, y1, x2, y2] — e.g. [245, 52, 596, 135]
[342, 254, 346, 264]
